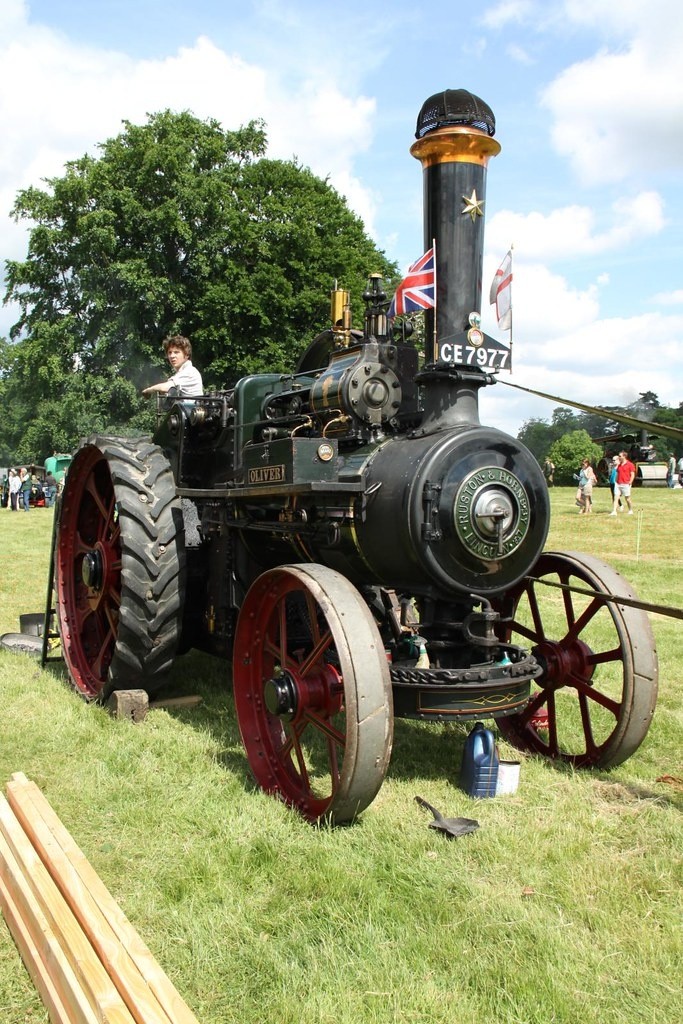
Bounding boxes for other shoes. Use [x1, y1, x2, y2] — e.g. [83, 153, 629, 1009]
[609, 510, 617, 515]
[628, 511, 633, 515]
[619, 505, 624, 512]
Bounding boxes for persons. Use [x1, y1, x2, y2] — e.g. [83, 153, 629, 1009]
[141, 336, 203, 417]
[667, 453, 676, 488]
[608, 456, 624, 510]
[44, 471, 57, 506]
[9, 467, 41, 511]
[676, 458, 683, 487]
[575, 458, 595, 513]
[542, 459, 555, 486]
[609, 451, 635, 515]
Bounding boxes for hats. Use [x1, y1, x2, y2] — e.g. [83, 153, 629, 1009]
[669, 452, 673, 456]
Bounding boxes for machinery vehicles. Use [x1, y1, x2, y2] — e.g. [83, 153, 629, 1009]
[588, 412, 678, 489]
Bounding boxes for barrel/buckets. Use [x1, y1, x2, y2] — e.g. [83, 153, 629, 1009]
[459, 722, 499, 799]
[496, 760, 521, 795]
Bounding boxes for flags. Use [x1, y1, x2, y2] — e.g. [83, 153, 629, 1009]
[387, 248, 435, 319]
[490, 249, 513, 329]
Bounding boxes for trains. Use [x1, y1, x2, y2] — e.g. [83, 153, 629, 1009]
[1, 85, 661, 830]
